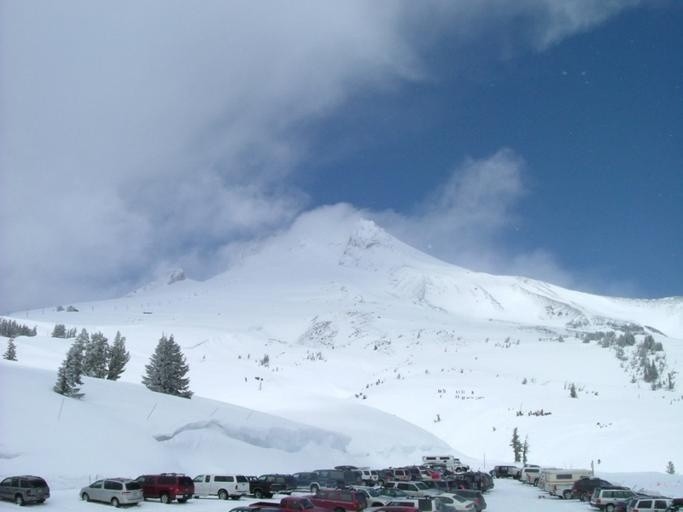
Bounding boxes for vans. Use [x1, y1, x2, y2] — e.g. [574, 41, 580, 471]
[79, 478, 144, 507]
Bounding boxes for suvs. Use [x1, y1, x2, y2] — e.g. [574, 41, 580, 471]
[0, 474, 49, 506]
